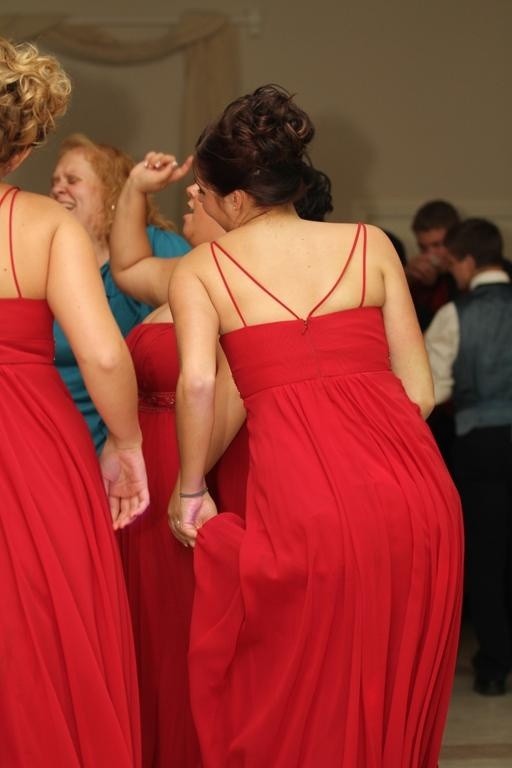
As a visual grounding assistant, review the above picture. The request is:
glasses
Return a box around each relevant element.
[418,241,443,253]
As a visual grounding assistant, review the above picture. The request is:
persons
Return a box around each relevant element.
[108,86,463,768]
[406,201,512,697]
[0,39,151,768]
[49,132,192,457]
